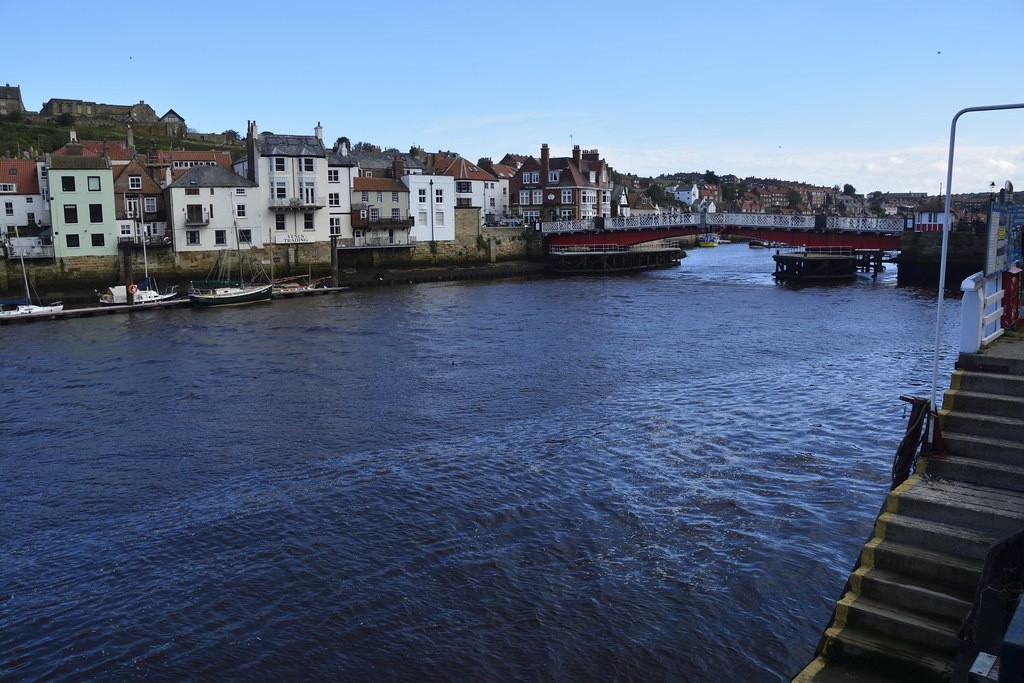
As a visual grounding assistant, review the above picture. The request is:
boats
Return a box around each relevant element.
[696,233,719,248]
[543,244,682,276]
[771,245,858,283]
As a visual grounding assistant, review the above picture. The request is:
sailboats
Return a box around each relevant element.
[0,226,64,318]
[99,193,179,307]
[187,192,272,308]
